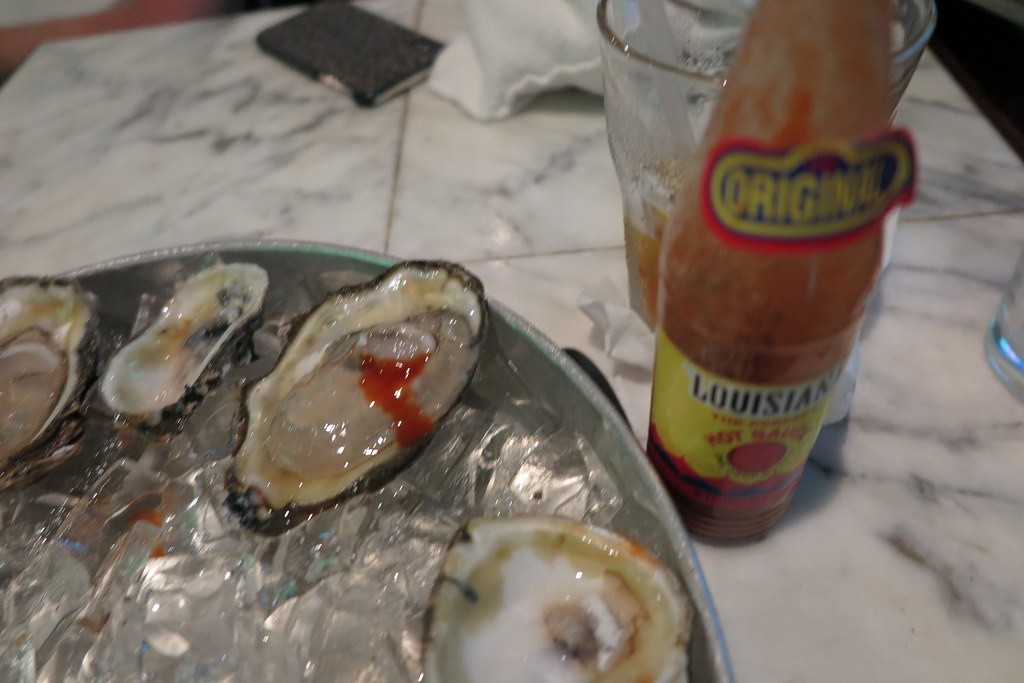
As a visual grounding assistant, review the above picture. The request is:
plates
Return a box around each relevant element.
[0,233,735,683]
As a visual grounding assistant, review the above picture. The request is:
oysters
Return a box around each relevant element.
[0,258,692,682]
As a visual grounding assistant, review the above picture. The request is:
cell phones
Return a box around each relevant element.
[256,0,447,104]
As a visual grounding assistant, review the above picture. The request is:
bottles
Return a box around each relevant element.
[646,0,884,545]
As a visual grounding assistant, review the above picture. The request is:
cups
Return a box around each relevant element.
[596,0,940,332]
[984,246,1024,406]
